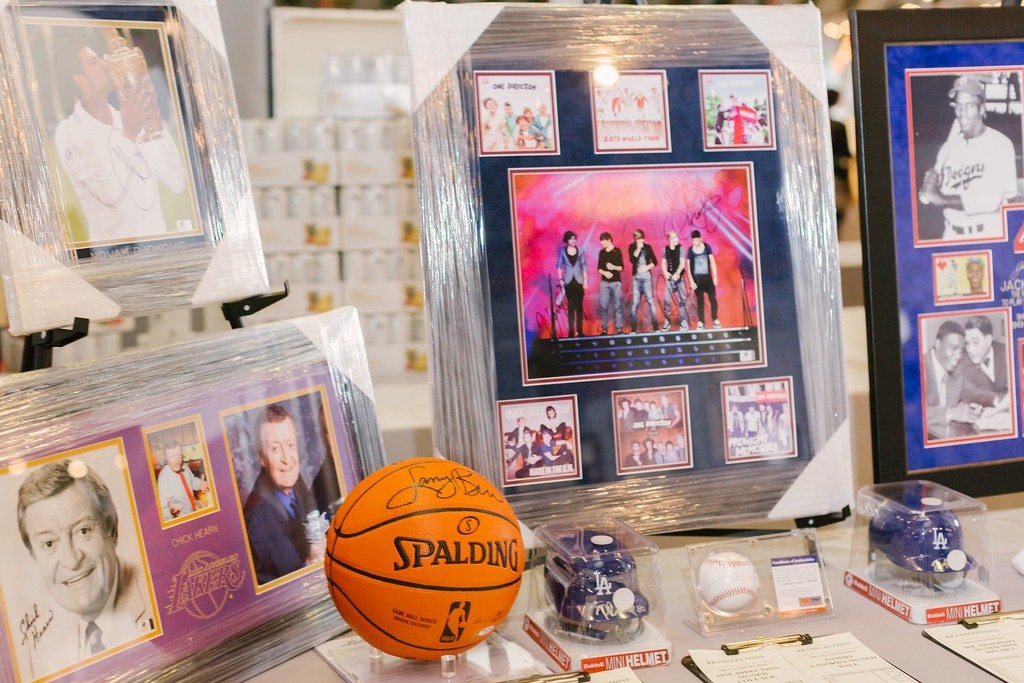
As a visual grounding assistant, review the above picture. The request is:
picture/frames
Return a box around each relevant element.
[847,6,1024,505]
[0,305,389,683]
[0,0,272,337]
[394,0,856,550]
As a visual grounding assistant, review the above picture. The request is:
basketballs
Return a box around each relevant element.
[326,458,524,664]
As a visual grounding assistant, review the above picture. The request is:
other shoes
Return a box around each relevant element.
[662,319,671,331]
[630,326,637,334]
[654,323,660,332]
[698,323,705,330]
[714,319,721,329]
[680,323,688,330]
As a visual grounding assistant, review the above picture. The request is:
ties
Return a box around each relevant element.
[178,471,196,511]
[85,622,105,655]
[291,499,305,524]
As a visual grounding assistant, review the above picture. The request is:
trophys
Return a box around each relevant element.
[102,25,165,141]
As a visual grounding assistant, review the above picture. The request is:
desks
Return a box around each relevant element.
[244,384,1024,683]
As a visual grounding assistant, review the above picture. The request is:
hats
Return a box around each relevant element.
[948,77,984,101]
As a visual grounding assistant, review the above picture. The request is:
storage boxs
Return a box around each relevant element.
[520,514,674,673]
[53,115,429,382]
[842,479,1002,627]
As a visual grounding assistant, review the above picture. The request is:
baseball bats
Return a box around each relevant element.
[920,116,962,205]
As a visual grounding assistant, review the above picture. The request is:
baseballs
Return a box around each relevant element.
[697,549,762,612]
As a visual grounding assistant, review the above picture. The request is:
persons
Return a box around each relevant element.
[960,314,1010,439]
[481,85,792,484]
[18,459,155,683]
[244,404,326,587]
[922,321,984,443]
[916,74,1018,238]
[52,40,191,243]
[156,438,211,523]
[965,256,986,295]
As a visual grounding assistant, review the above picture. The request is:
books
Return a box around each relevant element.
[971,406,1012,435]
